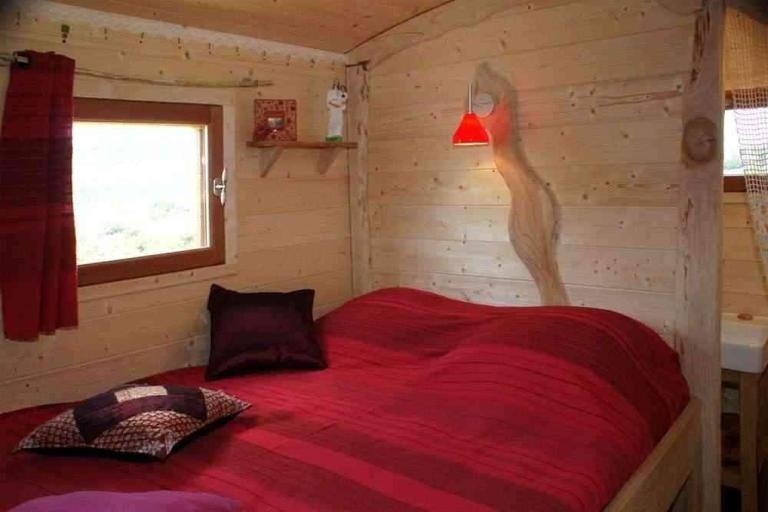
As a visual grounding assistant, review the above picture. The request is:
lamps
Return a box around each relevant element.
[452,80,490,146]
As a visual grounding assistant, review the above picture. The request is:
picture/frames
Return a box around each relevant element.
[254,99,298,143]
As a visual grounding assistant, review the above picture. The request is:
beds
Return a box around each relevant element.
[0,284,707,512]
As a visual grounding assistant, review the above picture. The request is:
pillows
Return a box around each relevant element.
[14,381,251,463]
[206,284,327,377]
